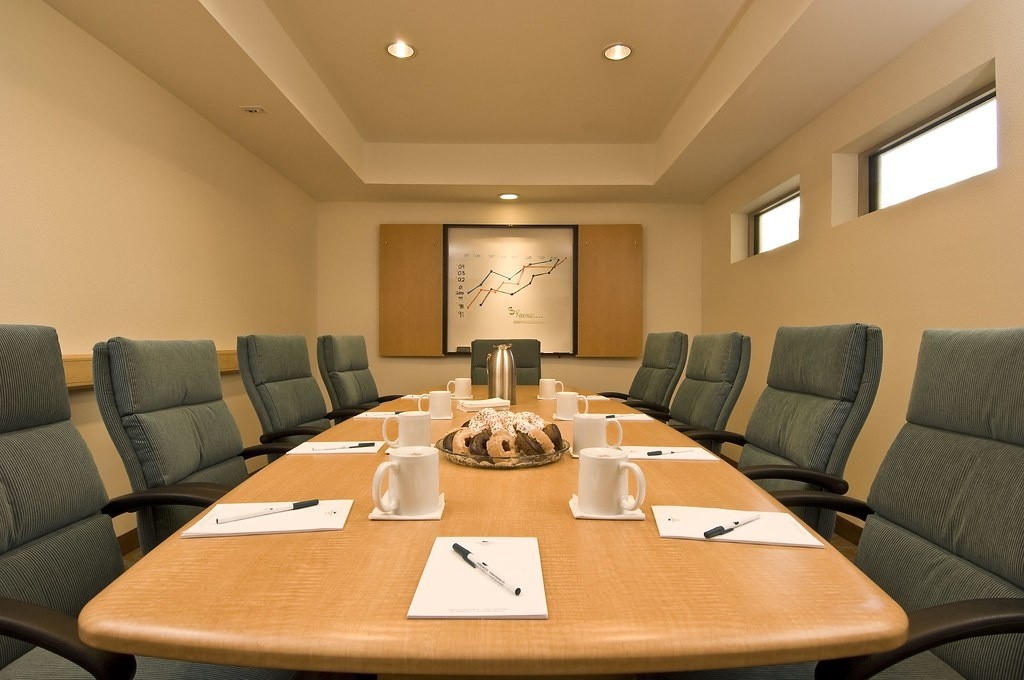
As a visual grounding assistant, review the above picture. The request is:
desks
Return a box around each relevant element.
[76,389,908,680]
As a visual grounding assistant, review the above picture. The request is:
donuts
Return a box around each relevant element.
[443,407,562,462]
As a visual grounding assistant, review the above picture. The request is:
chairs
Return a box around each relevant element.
[316,334,405,426]
[594,332,687,421]
[237,334,365,464]
[91,336,298,558]
[634,330,751,453]
[0,323,299,680]
[675,323,883,544]
[774,330,1024,680]
[470,338,542,387]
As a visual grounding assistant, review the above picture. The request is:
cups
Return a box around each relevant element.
[382,411,431,448]
[371,446,441,516]
[557,391,589,419]
[418,390,452,418]
[447,378,472,399]
[580,445,646,516]
[573,413,622,455]
[539,379,564,398]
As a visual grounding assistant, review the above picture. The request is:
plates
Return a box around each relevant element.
[435,436,571,469]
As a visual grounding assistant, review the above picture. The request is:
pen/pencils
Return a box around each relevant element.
[647,448,696,456]
[705,513,761,538]
[365,412,400,416]
[452,543,523,596]
[411,395,424,398]
[214,499,321,525]
[312,442,376,450]
[606,413,634,418]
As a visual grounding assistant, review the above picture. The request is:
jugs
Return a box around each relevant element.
[486,342,517,405]
